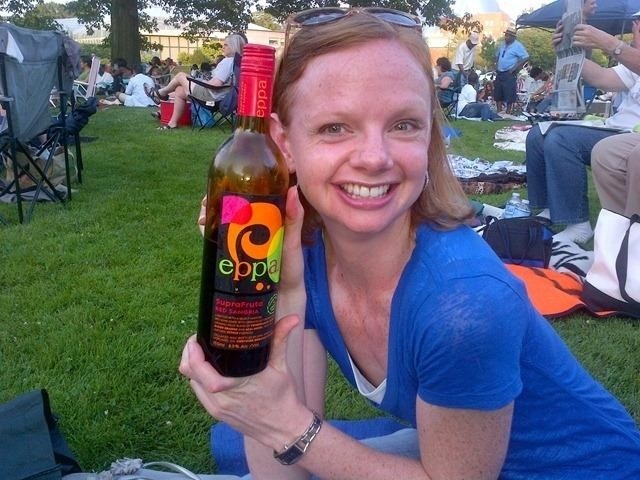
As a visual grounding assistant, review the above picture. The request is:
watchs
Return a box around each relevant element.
[611,41,625,59]
[272,406,323,465]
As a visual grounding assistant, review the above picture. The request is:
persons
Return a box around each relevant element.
[478,81,494,102]
[150,32,249,119]
[75,54,175,108]
[527,66,555,112]
[179,5,640,480]
[591,132,640,218]
[525,11,640,244]
[190,55,225,80]
[451,32,479,84]
[433,56,457,105]
[457,73,503,120]
[494,27,529,113]
[143,35,246,131]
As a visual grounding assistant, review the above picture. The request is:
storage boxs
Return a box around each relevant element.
[586,100,611,117]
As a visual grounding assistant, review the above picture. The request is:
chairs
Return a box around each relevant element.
[186,76,238,132]
[522,85,597,165]
[0,21,101,228]
[441,70,461,122]
[151,66,175,85]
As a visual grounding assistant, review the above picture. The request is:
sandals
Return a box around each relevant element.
[151,111,161,120]
[145,87,169,101]
[156,123,178,131]
[143,83,161,104]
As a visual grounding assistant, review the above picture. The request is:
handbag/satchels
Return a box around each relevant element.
[482,214,553,269]
[580,205,640,314]
[5,146,77,192]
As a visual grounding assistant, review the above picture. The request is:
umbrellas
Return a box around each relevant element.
[514,0,640,68]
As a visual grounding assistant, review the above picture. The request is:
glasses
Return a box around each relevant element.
[282,6,424,66]
[501,50,505,58]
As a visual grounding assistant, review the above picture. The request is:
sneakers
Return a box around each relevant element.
[551,220,594,245]
[535,208,551,220]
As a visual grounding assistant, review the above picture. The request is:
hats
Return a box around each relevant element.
[503,27,517,37]
[469,32,479,45]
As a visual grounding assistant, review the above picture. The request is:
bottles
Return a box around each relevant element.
[513,199,531,218]
[505,193,520,218]
[196,43,291,375]
